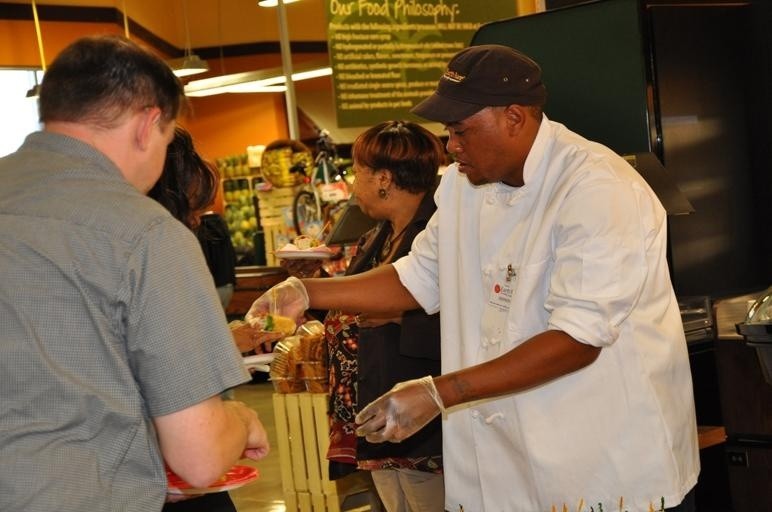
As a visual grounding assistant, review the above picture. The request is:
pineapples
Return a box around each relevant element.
[215,153,251,178]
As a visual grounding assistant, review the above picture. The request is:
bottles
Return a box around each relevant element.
[215,155,261,203]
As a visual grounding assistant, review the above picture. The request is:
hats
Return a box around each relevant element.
[408,43,549,124]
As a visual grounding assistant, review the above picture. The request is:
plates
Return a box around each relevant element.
[167,465,259,497]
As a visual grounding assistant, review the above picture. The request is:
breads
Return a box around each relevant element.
[270,320,329,392]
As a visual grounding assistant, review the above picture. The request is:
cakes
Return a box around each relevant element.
[279,236,330,251]
[252,313,296,334]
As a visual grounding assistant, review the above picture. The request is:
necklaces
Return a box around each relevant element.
[379,224,408,261]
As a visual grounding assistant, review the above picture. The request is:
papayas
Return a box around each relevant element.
[224,190,262,248]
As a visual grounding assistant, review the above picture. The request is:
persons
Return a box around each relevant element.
[323,119,447,512]
[143,120,237,511]
[1,35,270,511]
[244,42,705,512]
